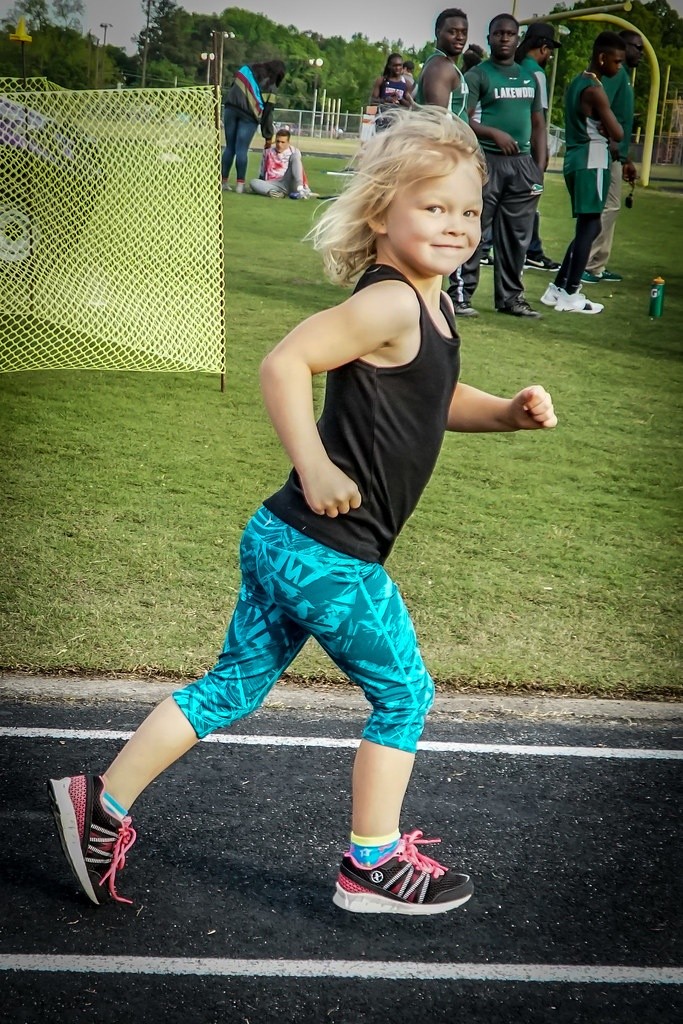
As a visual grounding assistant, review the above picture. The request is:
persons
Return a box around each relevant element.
[540,31,625,315]
[480,24,561,272]
[371,53,415,111]
[447,13,547,318]
[222,60,286,193]
[403,61,418,97]
[250,129,312,199]
[50,105,559,915]
[461,44,482,73]
[415,8,468,125]
[581,30,644,284]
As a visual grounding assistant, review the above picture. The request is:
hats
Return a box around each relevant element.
[272,60,286,87]
[525,23,562,50]
[402,61,414,69]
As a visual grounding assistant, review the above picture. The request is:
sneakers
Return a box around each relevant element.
[453,300,480,318]
[555,285,605,314]
[523,257,561,272]
[46,774,137,904]
[580,270,599,283]
[296,190,310,200]
[540,282,559,306]
[269,189,285,199]
[498,301,543,318]
[222,181,232,190]
[333,830,475,915]
[594,270,623,281]
[479,255,494,266]
[236,183,246,193]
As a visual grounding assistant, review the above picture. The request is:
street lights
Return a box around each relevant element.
[210,30,235,86]
[201,52,216,86]
[307,57,324,103]
[99,23,114,87]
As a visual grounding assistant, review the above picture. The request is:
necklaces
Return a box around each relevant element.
[584,70,601,83]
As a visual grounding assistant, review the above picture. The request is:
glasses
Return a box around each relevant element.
[628,42,645,52]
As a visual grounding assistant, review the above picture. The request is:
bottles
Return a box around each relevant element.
[649,276,665,317]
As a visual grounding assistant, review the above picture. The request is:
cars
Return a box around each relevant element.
[0,96,111,281]
